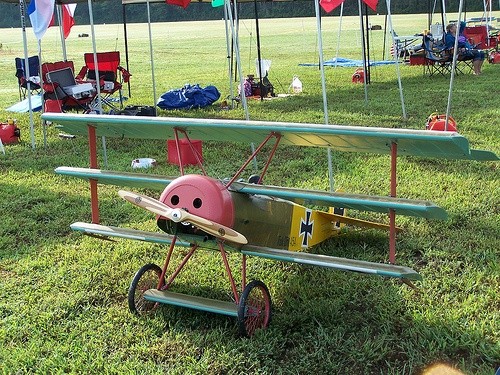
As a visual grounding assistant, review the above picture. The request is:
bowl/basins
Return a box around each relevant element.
[130,158,157,169]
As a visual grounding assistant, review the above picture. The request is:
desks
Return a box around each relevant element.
[395,35,427,61]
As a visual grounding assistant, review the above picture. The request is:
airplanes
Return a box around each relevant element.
[40,112,500,340]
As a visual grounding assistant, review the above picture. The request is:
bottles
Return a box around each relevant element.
[292,77,302,93]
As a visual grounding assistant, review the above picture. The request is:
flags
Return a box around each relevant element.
[62,4,78,39]
[212,0,225,7]
[27,0,55,41]
[319,0,343,13]
[165,0,190,8]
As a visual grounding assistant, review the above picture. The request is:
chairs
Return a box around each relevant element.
[15,52,133,116]
[421,30,500,79]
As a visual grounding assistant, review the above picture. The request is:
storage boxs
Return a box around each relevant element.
[168,139,202,164]
[410,54,433,65]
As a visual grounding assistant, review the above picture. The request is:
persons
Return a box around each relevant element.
[445,24,485,75]
